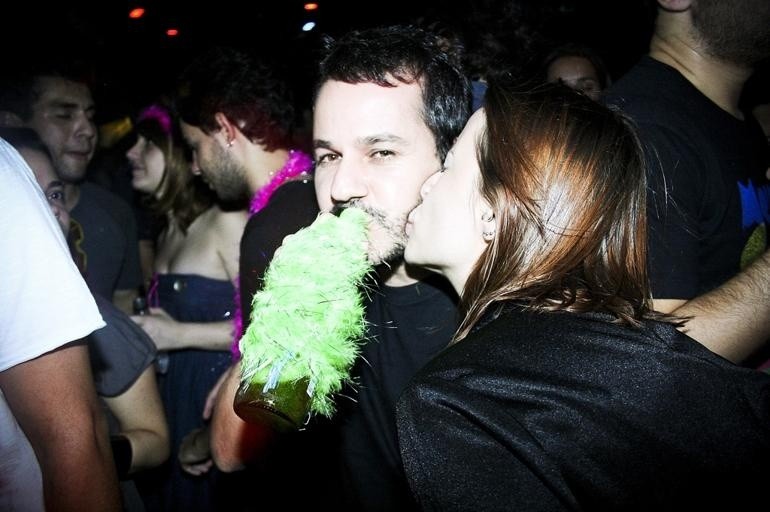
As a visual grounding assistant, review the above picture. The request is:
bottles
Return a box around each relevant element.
[132,297,170,375]
[232,375,314,438]
